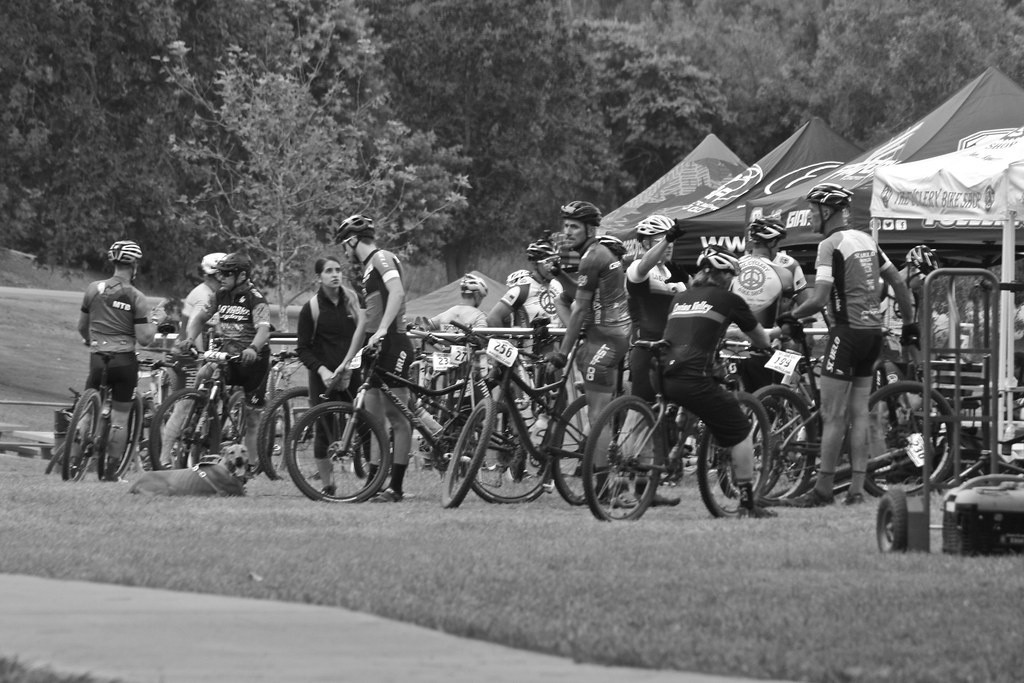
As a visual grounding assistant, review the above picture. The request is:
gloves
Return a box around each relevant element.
[900,323,919,346]
[775,312,798,328]
[666,218,687,242]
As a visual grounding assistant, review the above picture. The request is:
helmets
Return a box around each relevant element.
[526,238,562,261]
[595,235,628,255]
[460,274,488,298]
[506,270,532,288]
[805,183,854,207]
[108,240,143,263]
[906,245,941,275]
[696,245,741,276]
[201,253,227,275]
[636,214,675,242]
[560,200,602,226]
[216,253,252,279]
[747,218,787,244]
[335,215,375,244]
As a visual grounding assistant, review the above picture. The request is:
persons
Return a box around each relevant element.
[424,184,941,519]
[297,255,366,500]
[181,251,271,478]
[66,241,167,483]
[147,252,229,474]
[329,214,415,503]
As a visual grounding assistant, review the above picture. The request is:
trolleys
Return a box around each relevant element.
[876,268,1013,555]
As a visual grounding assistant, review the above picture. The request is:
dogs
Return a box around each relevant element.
[127,444,249,497]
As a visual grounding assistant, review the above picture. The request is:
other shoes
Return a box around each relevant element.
[635,479,682,506]
[737,508,779,521]
[611,493,636,509]
[842,492,866,507]
[780,489,835,509]
[320,487,336,499]
[102,474,130,483]
[508,448,526,483]
[370,489,403,503]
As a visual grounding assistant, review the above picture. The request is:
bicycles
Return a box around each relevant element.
[43,301,956,521]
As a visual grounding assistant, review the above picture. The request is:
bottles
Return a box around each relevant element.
[210,380,221,400]
[413,404,443,435]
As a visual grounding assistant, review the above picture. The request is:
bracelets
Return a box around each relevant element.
[248,344,258,352]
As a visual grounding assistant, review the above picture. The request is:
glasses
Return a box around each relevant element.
[341,234,358,251]
[221,270,236,277]
[537,256,561,265]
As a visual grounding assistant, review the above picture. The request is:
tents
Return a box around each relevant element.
[744,67,1024,440]
[606,115,865,270]
[549,133,751,273]
